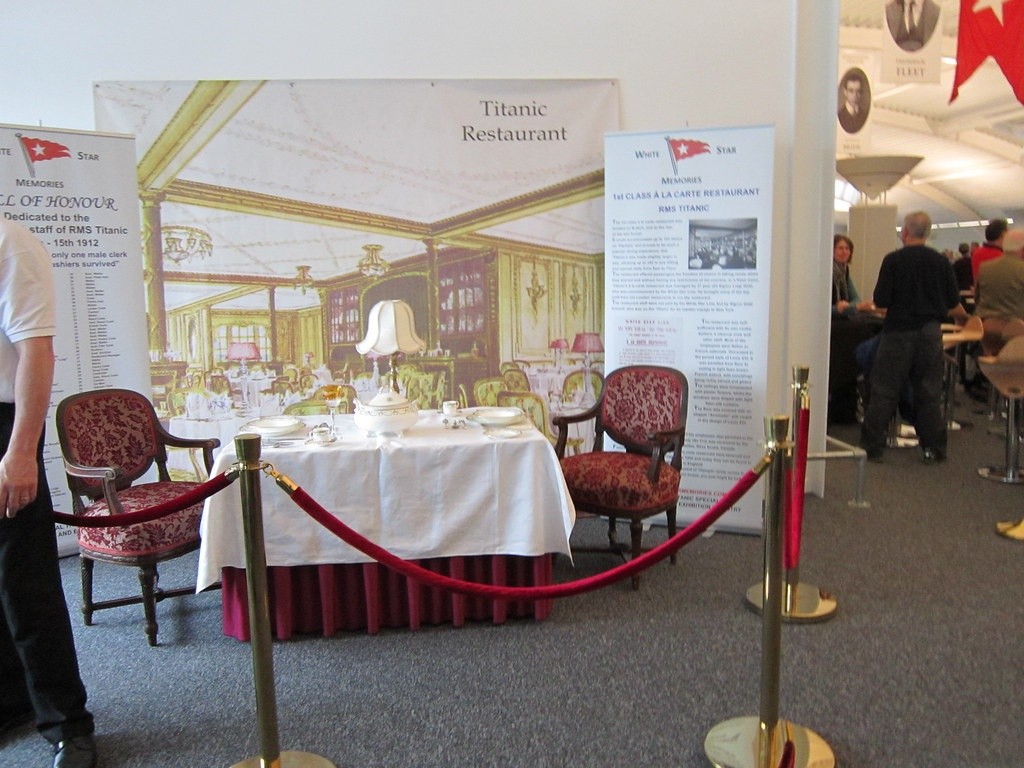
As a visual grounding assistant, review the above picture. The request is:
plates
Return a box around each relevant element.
[247,418,300,430]
[466,415,526,423]
[474,409,522,419]
[239,423,306,435]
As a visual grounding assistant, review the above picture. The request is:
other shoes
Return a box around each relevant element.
[868,450,884,463]
[924,445,947,465]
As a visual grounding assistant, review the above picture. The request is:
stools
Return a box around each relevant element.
[941,290,1024,484]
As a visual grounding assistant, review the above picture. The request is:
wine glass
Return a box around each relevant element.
[323,385,342,437]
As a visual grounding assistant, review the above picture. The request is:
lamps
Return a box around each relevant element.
[224,341,261,406]
[140,225,214,266]
[291,266,314,296]
[548,338,570,373]
[571,332,606,411]
[351,298,427,440]
[356,242,390,277]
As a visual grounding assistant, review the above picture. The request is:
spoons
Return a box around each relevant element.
[443,418,449,429]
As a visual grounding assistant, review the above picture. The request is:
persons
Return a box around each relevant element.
[886,0,940,51]
[0,215,95,768]
[689,234,758,269]
[833,213,1024,464]
[838,75,865,132]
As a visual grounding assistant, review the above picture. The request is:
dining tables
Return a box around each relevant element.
[169,409,251,478]
[292,366,332,386]
[320,379,421,404]
[223,369,277,402]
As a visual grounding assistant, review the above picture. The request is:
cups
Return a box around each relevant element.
[443,401,459,415]
[309,426,330,445]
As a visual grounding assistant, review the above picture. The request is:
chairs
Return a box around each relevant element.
[460,358,585,459]
[552,366,690,587]
[167,364,395,414]
[56,389,221,646]
[563,369,607,457]
[406,369,446,413]
[195,407,578,645]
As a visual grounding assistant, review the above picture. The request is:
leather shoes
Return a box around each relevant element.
[52,731,97,768]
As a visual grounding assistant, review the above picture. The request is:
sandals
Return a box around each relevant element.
[995,519,1024,542]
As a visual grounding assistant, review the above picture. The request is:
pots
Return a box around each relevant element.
[352,383,419,436]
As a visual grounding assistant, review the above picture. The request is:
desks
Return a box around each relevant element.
[452,357,489,408]
[844,307,921,451]
